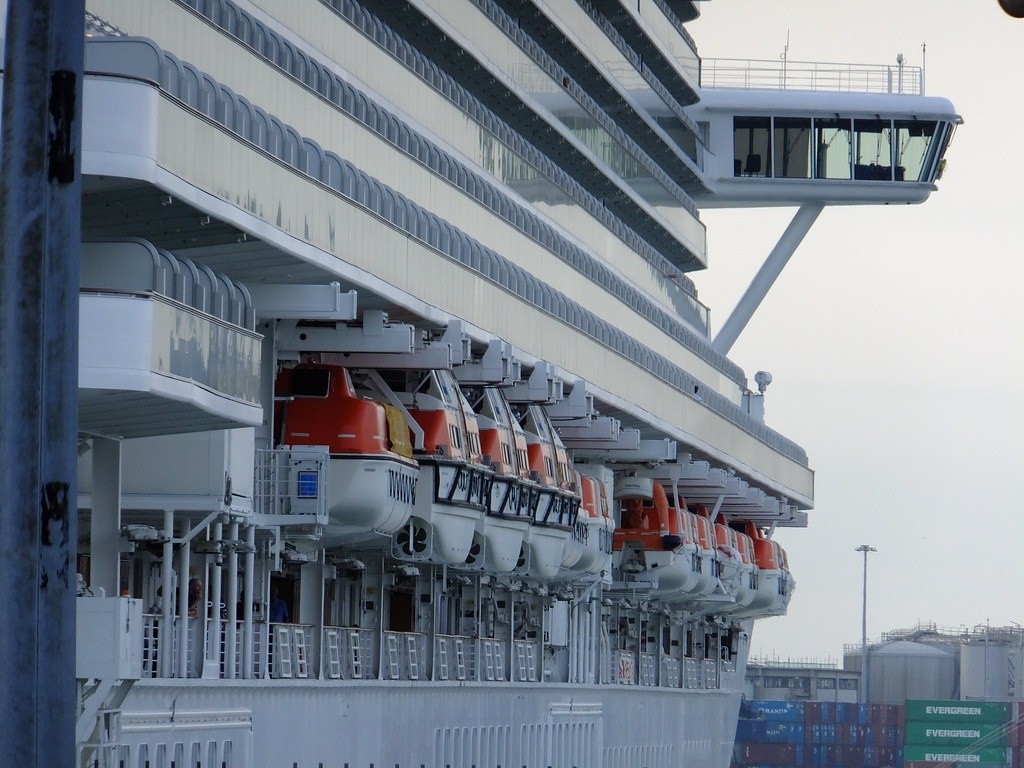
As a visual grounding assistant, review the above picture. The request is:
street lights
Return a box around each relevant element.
[856,546,879,701]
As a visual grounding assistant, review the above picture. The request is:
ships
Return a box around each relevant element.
[0,0,963,766]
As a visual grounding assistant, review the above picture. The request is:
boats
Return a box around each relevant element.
[464,384,540,579]
[613,480,796,623]
[553,472,615,598]
[358,369,498,570]
[513,403,582,586]
[272,367,420,554]
